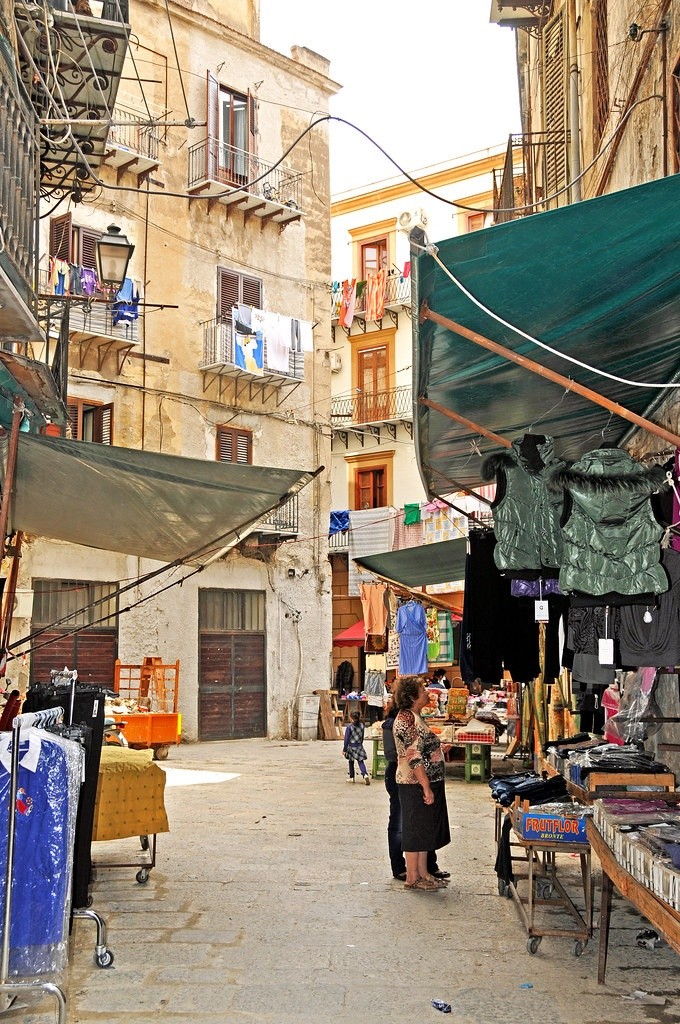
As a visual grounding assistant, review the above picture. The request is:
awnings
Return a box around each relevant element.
[350,530,468,619]
[332,604,463,648]
[5,427,321,659]
[408,172,680,531]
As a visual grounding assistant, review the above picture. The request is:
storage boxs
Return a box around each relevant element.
[510,807,590,845]
[423,686,495,744]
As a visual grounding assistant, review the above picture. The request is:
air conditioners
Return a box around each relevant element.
[330,352,342,372]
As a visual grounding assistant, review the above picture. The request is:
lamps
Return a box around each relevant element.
[39,221,136,317]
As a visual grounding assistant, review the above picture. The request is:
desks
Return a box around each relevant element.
[340,699,368,726]
[372,737,487,783]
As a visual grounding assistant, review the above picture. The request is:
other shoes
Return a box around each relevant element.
[428,869,450,878]
[363,774,370,785]
[346,777,355,783]
[395,873,407,881]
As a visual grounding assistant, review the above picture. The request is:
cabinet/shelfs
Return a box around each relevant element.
[492,755,680,983]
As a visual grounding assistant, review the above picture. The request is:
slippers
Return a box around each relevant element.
[404,872,448,891]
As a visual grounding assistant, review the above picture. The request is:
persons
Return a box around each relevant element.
[430,668,451,689]
[368,705,384,726]
[343,710,370,785]
[383,673,451,890]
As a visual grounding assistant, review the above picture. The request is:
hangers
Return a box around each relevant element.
[28,669,104,697]
[5,706,82,741]
[599,428,618,449]
